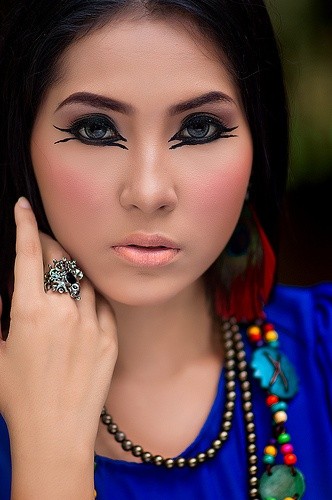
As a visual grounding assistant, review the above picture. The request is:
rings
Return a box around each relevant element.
[44,257,84,301]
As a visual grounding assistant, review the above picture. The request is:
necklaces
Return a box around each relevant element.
[100,318,259,500]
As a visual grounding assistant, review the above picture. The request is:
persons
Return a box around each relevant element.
[0,0,332,500]
